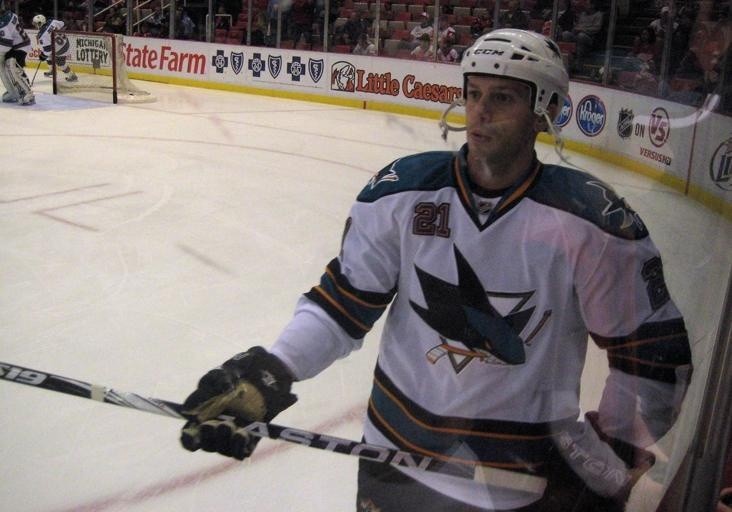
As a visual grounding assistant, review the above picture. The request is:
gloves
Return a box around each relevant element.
[514,410,657,512]
[179,346,297,462]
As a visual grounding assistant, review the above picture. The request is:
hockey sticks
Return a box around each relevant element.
[29,60,42,87]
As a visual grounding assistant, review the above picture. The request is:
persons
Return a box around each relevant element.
[178,26,692,511]
[63,0,386,55]
[0,10,36,106]
[410,0,732,112]
[32,15,77,81]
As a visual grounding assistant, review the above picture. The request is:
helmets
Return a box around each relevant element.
[31,14,47,28]
[460,26,570,117]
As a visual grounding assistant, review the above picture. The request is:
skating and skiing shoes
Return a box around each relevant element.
[65,73,78,82]
[2,92,18,101]
[17,92,35,104]
[44,70,56,78]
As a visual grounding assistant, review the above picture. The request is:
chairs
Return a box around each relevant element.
[1,0,732,112]
